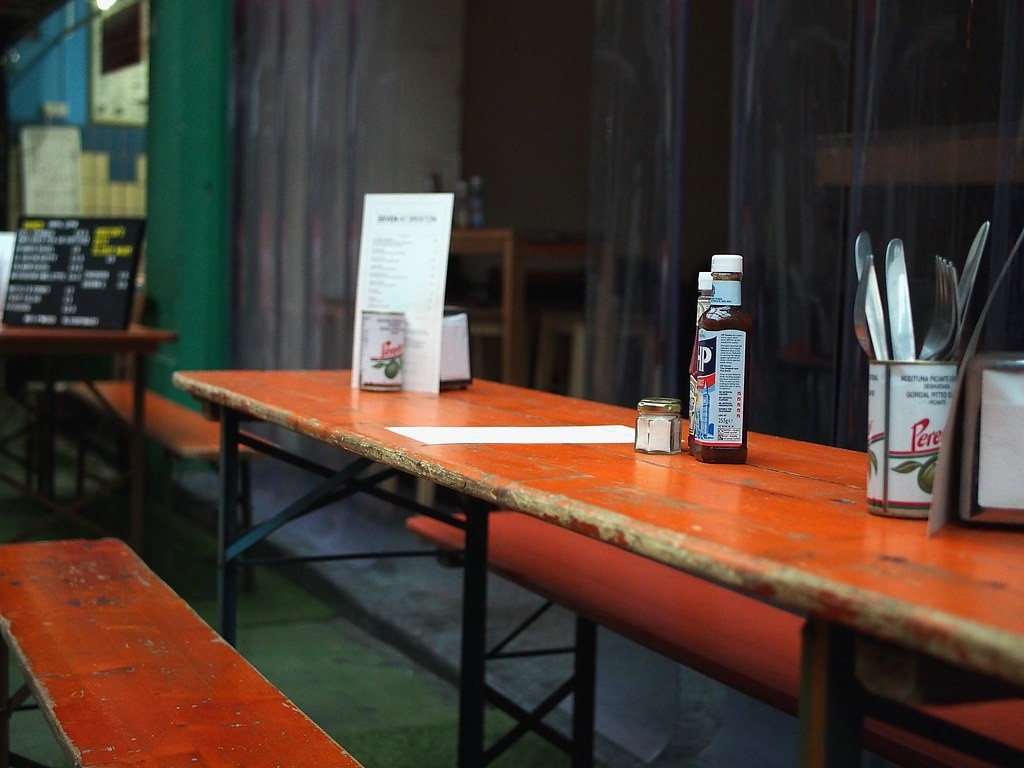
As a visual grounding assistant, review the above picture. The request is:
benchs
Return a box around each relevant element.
[60,380,277,462]
[0,543,372,768]
[404,507,1024,768]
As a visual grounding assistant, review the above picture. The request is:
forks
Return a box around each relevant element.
[931,262,961,360]
[853,254,876,360]
[918,253,953,360]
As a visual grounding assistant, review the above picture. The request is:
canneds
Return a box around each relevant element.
[359,310,406,392]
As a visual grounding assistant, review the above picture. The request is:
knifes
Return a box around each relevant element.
[854,230,889,361]
[959,222,991,325]
[885,238,917,361]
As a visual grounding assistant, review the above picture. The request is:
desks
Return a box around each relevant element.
[0,323,177,555]
[175,359,1024,768]
[452,224,596,386]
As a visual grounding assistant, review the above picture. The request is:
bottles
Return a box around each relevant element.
[693,255,752,464]
[688,272,712,458]
[634,398,684,455]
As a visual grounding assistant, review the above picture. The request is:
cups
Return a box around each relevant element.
[864,360,959,520]
[358,309,406,392]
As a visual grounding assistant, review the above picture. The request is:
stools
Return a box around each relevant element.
[534,310,664,407]
